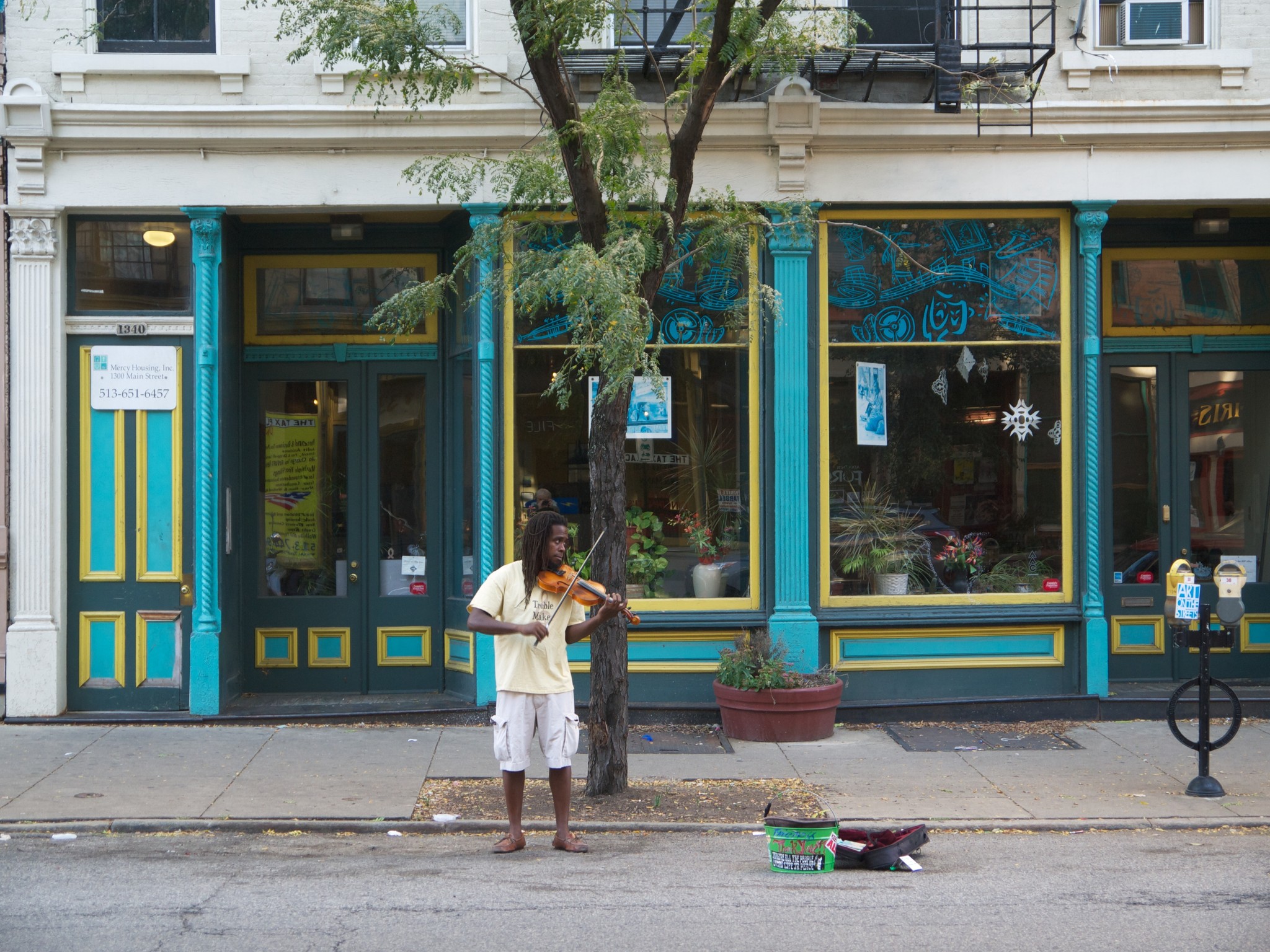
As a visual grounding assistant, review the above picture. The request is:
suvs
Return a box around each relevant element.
[683,503,961,598]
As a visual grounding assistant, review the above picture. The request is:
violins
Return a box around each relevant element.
[538,564,641,626]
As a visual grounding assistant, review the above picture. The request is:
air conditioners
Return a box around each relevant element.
[1116,0,1191,47]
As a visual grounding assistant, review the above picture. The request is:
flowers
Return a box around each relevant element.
[931,526,987,573]
[669,512,731,564]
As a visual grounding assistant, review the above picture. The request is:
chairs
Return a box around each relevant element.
[982,537,1000,554]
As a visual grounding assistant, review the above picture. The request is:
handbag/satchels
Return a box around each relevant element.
[876,420,885,435]
[865,409,884,431]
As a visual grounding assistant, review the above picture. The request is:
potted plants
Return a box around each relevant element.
[713,629,845,742]
[516,422,750,597]
[832,476,1045,595]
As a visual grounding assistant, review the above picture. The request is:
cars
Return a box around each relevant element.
[1034,540,1234,592]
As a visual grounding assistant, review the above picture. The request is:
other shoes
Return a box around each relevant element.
[860,413,868,421]
[552,833,589,852]
[492,834,526,852]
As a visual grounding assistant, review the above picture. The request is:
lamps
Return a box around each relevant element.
[329,214,364,242]
[141,220,177,248]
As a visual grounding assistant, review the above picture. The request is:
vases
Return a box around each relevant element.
[692,563,722,598]
[951,572,969,592]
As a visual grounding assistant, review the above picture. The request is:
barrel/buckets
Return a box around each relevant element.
[763,790,841,874]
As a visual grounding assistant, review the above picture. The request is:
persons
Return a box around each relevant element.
[467,512,629,854]
[525,488,560,518]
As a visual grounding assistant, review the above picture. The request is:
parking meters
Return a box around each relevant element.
[1162,557,1244,797]
